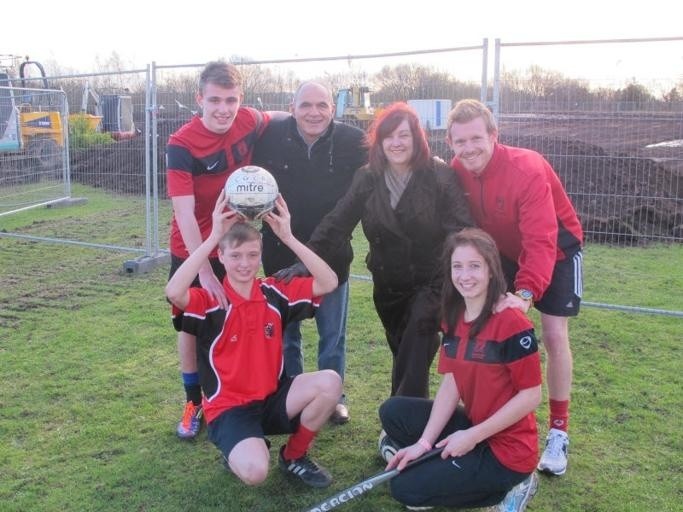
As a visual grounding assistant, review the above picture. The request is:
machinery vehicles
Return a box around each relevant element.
[334,84,381,124]
[78,85,136,140]
[0,59,103,186]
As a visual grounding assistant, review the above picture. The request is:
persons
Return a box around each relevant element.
[269,101,476,397]
[442,96,584,477]
[164,188,344,488]
[162,61,292,442]
[378,226,542,511]
[248,73,372,425]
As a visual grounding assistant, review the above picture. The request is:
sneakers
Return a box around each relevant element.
[494,472,537,512]
[536,428,569,476]
[177,401,202,438]
[331,404,350,423]
[278,445,333,488]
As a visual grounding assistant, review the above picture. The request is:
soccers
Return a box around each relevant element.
[224,165,277,221]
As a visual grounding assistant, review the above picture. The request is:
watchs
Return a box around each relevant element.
[514,288,535,309]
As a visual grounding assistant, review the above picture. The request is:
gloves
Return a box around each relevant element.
[273,262,309,285]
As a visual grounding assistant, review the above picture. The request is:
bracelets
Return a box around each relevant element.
[415,438,433,452]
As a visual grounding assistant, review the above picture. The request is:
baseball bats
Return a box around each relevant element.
[306,445,447,511]
